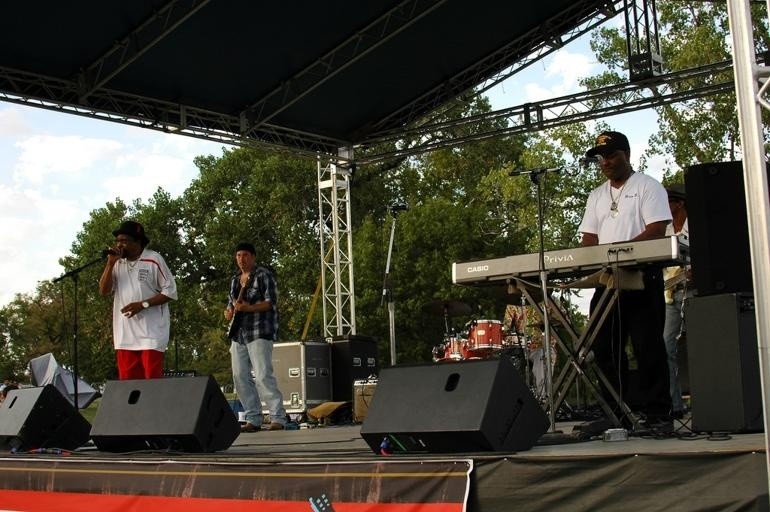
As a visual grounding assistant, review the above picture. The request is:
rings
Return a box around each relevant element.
[128,311,132,315]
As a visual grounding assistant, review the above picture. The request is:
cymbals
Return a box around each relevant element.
[497,281,553,305]
[422,300,475,316]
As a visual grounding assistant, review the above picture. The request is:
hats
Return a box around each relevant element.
[666,183,686,199]
[113,221,150,244]
[586,131,629,158]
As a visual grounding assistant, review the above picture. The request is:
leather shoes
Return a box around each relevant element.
[271,422,282,430]
[241,423,261,432]
[672,410,684,419]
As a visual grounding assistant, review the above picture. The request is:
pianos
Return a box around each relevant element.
[451,235,680,286]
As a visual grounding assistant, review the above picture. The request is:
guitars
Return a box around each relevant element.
[226,274,253,341]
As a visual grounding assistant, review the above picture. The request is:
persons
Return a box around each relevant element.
[222,243,288,433]
[661,181,698,418]
[501,294,561,401]
[0,378,22,405]
[571,130,673,438]
[98,220,179,381]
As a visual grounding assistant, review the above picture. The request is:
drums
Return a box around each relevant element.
[468,319,502,350]
[460,339,491,359]
[444,335,461,361]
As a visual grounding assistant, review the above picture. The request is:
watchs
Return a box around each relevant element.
[141,300,150,310]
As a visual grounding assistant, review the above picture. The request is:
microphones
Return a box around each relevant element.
[580,156,601,161]
[94,250,117,256]
[389,204,410,210]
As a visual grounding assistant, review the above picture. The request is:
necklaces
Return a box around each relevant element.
[609,171,629,211]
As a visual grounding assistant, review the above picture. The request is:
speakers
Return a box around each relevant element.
[0,385,92,451]
[359,356,554,452]
[684,162,769,297]
[89,374,241,453]
[685,293,763,434]
